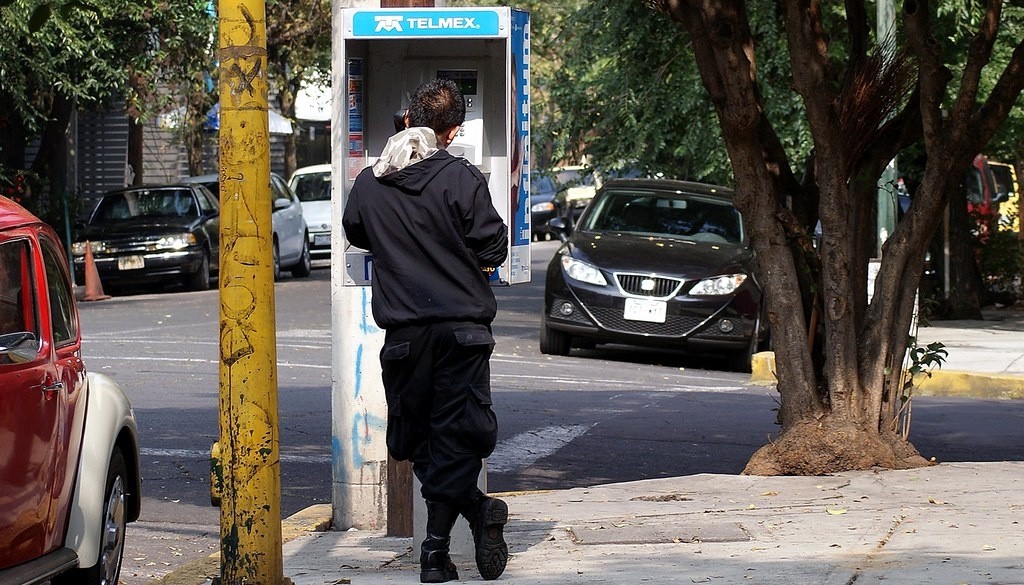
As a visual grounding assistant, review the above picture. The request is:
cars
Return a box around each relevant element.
[550,164,604,225]
[0,194,144,585]
[175,171,312,280]
[71,184,220,293]
[896,157,1022,260]
[529,171,570,238]
[608,159,685,179]
[540,177,773,372]
[285,163,331,256]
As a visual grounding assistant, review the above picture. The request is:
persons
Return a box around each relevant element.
[342,78,509,583]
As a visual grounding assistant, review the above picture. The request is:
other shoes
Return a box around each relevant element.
[470,496,507,580]
[421,536,458,583]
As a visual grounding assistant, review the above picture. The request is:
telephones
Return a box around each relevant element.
[393,109,406,134]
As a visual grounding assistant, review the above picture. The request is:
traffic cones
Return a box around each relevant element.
[78,239,112,301]
